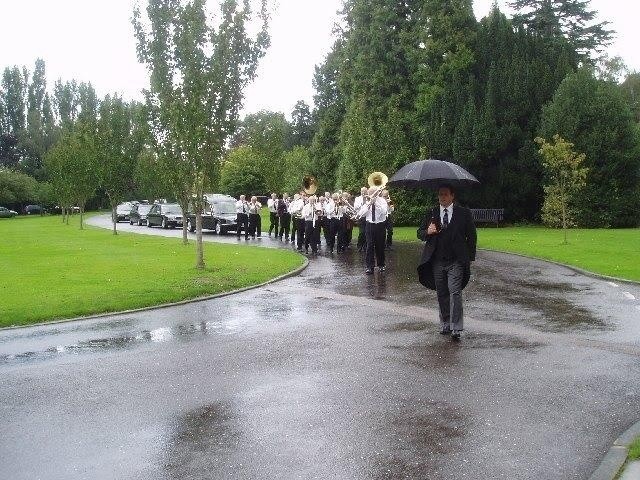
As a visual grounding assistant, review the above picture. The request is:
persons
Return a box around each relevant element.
[416,183,477,342]
[235,186,395,275]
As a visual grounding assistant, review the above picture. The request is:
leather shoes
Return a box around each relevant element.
[440,326,450,333]
[365,267,373,274]
[452,330,460,339]
[380,266,385,272]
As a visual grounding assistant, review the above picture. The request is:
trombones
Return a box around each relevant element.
[312,197,321,228]
[356,189,381,220]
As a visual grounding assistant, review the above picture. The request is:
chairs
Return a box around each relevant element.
[470,209,505,228]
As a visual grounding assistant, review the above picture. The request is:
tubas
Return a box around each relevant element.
[299,174,317,196]
[368,171,388,191]
[339,194,356,221]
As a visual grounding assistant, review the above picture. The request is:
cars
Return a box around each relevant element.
[0,206,20,218]
[47,203,80,214]
[111,204,131,222]
[129,204,151,226]
[122,198,166,204]
[149,202,184,229]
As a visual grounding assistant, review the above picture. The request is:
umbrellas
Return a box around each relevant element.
[385,159,482,234]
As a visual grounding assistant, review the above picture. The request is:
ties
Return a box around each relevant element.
[443,208,449,228]
[372,203,375,221]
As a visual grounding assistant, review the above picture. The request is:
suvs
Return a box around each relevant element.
[23,205,48,215]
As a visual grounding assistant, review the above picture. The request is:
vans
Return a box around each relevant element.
[186,192,250,234]
